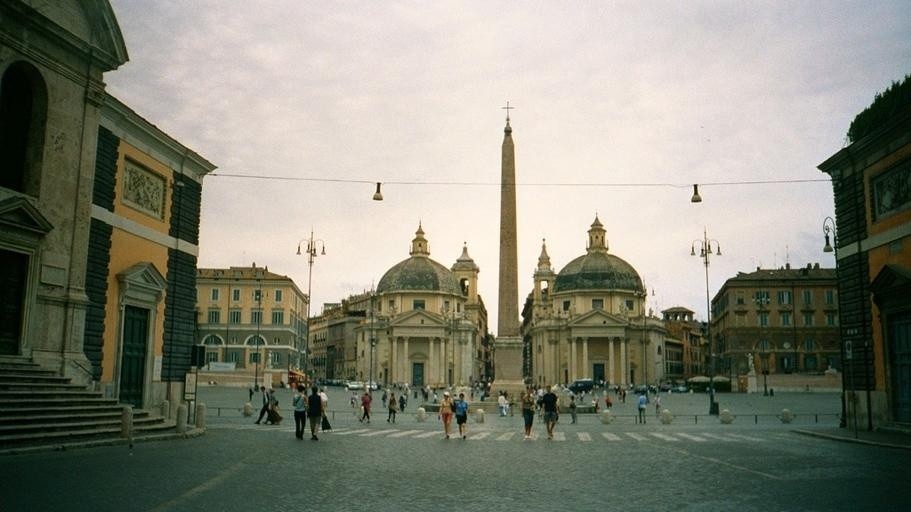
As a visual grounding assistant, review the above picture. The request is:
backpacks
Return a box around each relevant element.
[321,416,331,431]
[275,400,279,407]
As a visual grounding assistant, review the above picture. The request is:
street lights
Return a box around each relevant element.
[298,230,326,393]
[821,214,848,430]
[251,284,269,392]
[691,225,723,416]
[751,289,774,397]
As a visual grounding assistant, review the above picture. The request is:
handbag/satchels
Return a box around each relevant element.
[463,410,467,420]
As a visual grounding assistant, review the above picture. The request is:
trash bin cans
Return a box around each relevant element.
[713,401,719,415]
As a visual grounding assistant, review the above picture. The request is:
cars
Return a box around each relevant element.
[634,381,686,394]
[322,377,377,391]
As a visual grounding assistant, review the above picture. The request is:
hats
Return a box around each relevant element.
[442,390,451,396]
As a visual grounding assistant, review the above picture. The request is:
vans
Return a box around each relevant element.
[569,379,593,393]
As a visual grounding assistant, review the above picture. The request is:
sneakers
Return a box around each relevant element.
[387,418,396,423]
[294,431,319,441]
[359,418,371,424]
[255,421,280,425]
[442,434,468,441]
[523,433,555,441]
